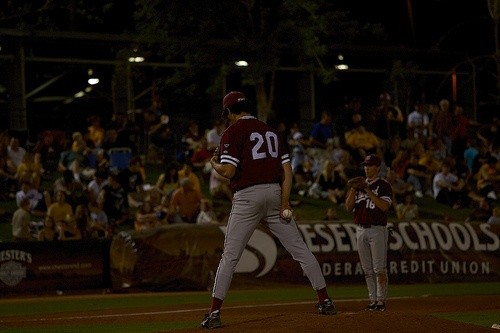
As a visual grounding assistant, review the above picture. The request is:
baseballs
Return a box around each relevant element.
[283,209,292,218]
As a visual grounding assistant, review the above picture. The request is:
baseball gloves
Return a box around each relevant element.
[347,177,366,189]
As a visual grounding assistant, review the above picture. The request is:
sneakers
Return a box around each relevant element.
[377,302,385,311]
[200,311,222,328]
[367,302,377,311]
[316,298,335,315]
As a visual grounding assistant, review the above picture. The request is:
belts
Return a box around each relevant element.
[356,222,386,227]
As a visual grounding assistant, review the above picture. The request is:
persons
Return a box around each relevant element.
[11,198,35,242]
[429,99,452,135]
[207,119,226,148]
[156,163,179,199]
[127,184,145,217]
[446,153,471,178]
[1,153,18,196]
[107,112,137,154]
[345,154,393,310]
[363,133,449,177]
[7,135,27,165]
[140,110,174,151]
[51,220,83,240]
[200,90,337,328]
[101,173,129,224]
[141,144,167,165]
[84,202,108,238]
[14,181,49,210]
[285,122,311,160]
[464,198,500,224]
[135,202,157,229]
[47,190,73,223]
[152,196,170,225]
[321,207,338,220]
[96,175,132,222]
[447,118,498,156]
[37,216,56,241]
[310,110,334,147]
[396,191,420,219]
[450,105,469,134]
[338,151,356,180]
[147,186,161,204]
[75,205,88,231]
[372,91,403,121]
[124,156,145,189]
[312,158,336,202]
[15,151,41,186]
[37,115,117,171]
[387,172,414,210]
[325,138,344,160]
[86,171,107,205]
[209,164,234,202]
[169,177,208,223]
[52,170,82,203]
[408,99,428,134]
[431,163,468,207]
[184,118,216,166]
[344,114,380,146]
[475,157,500,198]
[178,160,200,195]
[290,132,307,188]
[198,199,216,222]
[341,94,372,130]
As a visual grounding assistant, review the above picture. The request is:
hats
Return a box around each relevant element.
[361,154,381,166]
[222,91,248,120]
